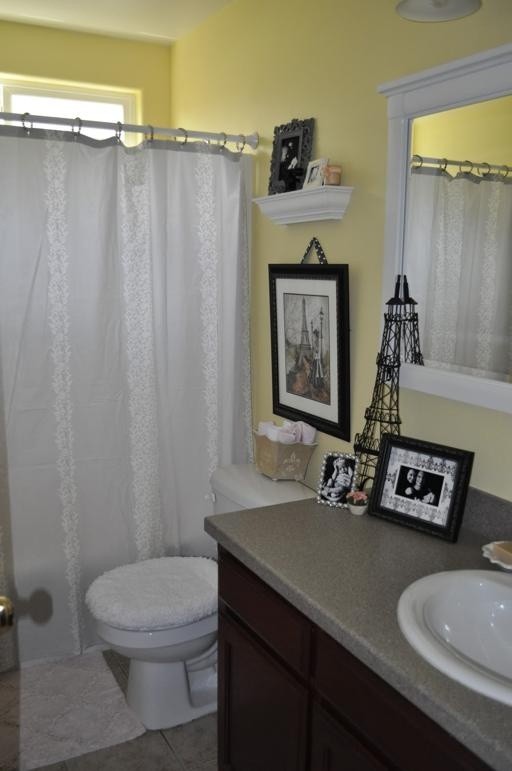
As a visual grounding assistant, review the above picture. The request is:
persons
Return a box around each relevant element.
[284,140,298,170]
[321,458,354,503]
[409,472,436,505]
[402,469,416,496]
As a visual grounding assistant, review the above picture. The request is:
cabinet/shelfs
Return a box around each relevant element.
[220,547,490,770]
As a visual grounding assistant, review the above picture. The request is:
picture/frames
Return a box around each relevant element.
[267,117,328,195]
[366,432,475,545]
[317,452,358,508]
[266,263,351,443]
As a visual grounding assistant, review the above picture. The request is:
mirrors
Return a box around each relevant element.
[379,38,511,413]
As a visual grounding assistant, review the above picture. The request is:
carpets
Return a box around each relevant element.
[18,651,147,771]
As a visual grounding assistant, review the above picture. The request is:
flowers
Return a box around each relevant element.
[346,490,370,505]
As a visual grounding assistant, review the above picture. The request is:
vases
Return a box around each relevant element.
[347,503,368,516]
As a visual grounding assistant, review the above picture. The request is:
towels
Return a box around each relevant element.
[257,420,316,448]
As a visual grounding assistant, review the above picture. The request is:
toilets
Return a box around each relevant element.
[83,467,318,734]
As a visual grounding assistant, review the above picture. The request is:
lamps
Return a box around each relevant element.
[393,0,483,27]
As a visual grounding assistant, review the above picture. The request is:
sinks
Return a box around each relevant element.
[396,566,512,710]
